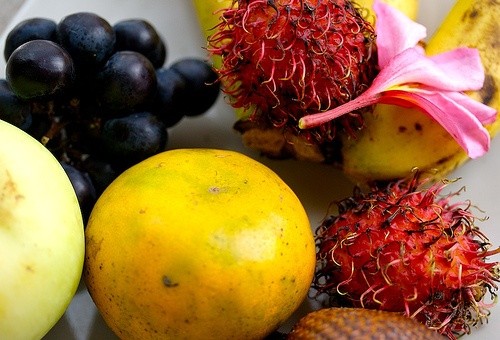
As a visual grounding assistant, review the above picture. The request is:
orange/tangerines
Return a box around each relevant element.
[85,148,316,340]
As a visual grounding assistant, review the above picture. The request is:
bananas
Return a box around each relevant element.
[191,0,500,186]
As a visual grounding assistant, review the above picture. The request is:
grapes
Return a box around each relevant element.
[0,10,222,218]
